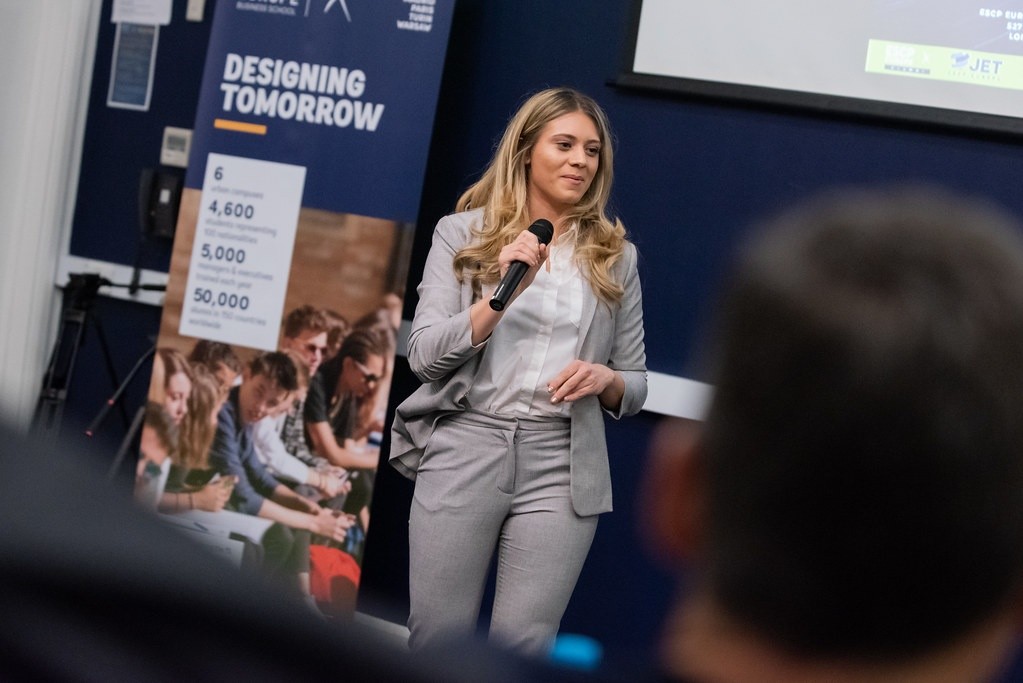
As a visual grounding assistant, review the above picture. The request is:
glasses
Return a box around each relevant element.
[353,359,383,383]
[291,337,329,356]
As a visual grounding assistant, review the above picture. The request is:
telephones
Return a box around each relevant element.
[137,166,185,249]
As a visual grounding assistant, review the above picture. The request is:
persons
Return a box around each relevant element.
[134,297,404,627]
[618,178,1023,683]
[388,84,647,665]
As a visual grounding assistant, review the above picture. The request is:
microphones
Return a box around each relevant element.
[489,218,554,312]
